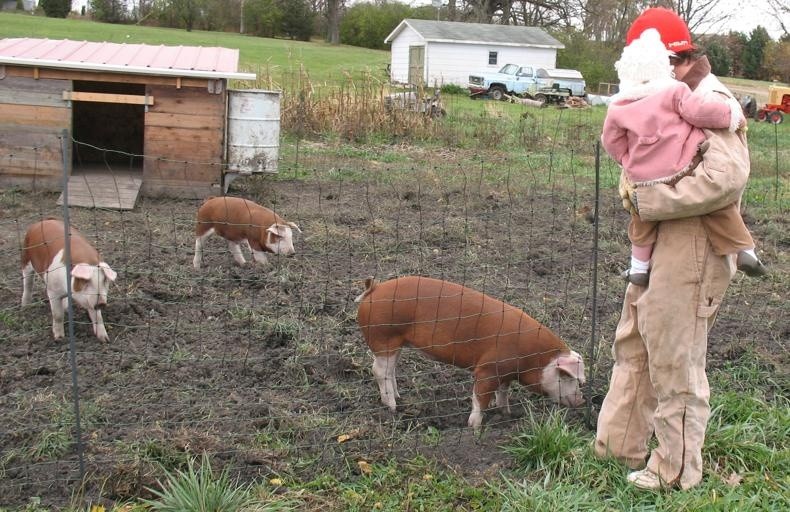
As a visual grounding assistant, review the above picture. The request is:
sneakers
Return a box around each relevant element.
[737,250,767,278]
[625,469,675,492]
[619,268,649,287]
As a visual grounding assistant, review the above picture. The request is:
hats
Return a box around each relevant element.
[615,29,672,84]
[627,7,697,51]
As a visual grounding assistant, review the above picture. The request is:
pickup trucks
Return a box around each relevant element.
[468,63,586,107]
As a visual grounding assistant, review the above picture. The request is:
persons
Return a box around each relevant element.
[575,7,751,491]
[600,28,765,286]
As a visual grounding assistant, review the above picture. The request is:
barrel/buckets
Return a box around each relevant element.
[226,90,282,173]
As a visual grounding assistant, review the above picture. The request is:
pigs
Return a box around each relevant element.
[192,194,302,270]
[18,216,119,344]
[353,273,587,434]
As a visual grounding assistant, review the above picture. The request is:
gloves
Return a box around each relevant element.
[618,171,637,216]
